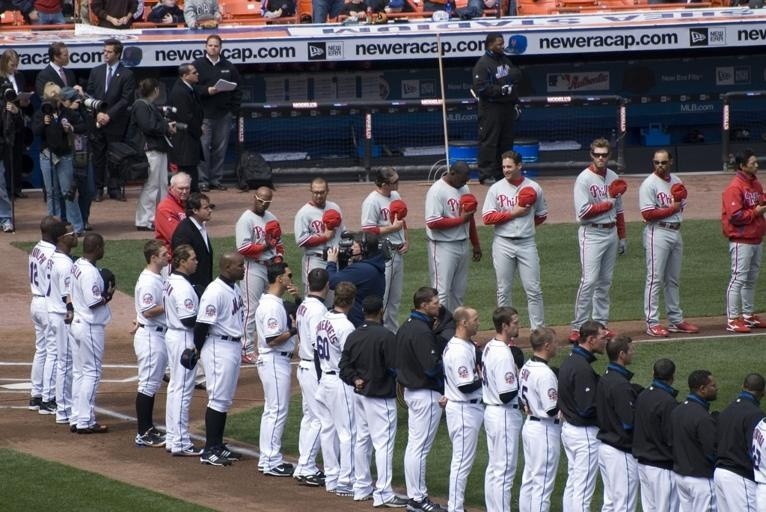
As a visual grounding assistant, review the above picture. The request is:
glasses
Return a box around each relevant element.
[255,195,272,205]
[57,232,75,240]
[654,160,668,165]
[591,152,609,158]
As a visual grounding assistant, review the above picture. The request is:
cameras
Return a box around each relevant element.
[42,100,57,113]
[170,120,187,133]
[1,82,16,102]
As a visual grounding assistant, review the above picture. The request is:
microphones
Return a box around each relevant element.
[351,251,362,257]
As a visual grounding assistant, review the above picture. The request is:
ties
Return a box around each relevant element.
[107,66,113,90]
[58,68,68,87]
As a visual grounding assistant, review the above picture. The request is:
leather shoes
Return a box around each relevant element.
[94,186,126,200]
[201,182,226,191]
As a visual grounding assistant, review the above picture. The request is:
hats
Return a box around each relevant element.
[460,193,476,216]
[265,220,282,241]
[390,201,406,224]
[324,209,341,230]
[672,182,688,202]
[610,180,627,198]
[519,186,537,207]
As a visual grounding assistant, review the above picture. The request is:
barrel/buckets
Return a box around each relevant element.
[447,140,482,181]
[513,136,538,178]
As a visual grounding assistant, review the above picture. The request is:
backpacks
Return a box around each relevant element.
[234,151,274,190]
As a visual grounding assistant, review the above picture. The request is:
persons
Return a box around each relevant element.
[1,0,766,511]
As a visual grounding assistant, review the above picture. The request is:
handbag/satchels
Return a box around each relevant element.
[104,140,150,182]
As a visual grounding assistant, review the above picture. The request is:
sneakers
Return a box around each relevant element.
[134,427,242,467]
[242,352,258,365]
[745,316,766,328]
[668,322,699,332]
[647,326,668,336]
[0,218,14,231]
[29,397,108,434]
[570,330,580,343]
[259,461,446,512]
[606,330,617,340]
[726,319,751,332]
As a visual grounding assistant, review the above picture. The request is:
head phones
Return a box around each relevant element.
[361,232,368,257]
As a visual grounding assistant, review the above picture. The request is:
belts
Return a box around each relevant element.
[496,404,519,410]
[139,324,169,332]
[504,236,525,239]
[591,223,617,228]
[281,351,293,358]
[471,398,482,404]
[326,371,336,375]
[658,222,681,230]
[208,333,241,342]
[316,253,322,256]
[529,416,560,424]
